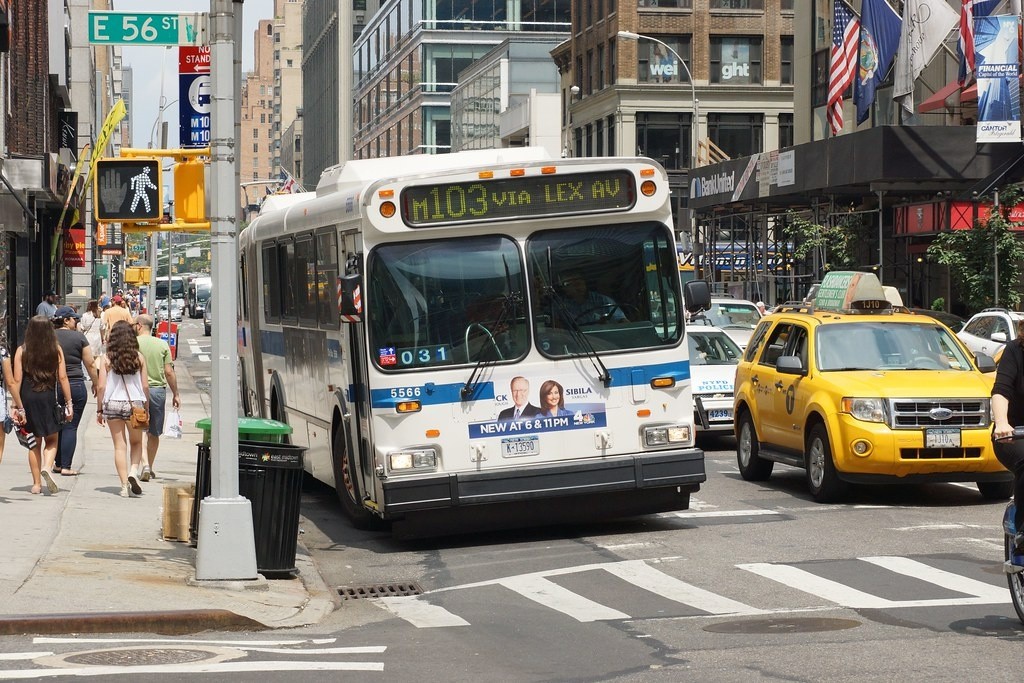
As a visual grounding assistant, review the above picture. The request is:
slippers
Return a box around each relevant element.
[53,469,61,473]
[62,470,80,475]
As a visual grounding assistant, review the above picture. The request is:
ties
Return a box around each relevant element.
[514,409,520,420]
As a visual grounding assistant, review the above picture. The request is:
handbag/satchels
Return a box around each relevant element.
[16,427,36,449]
[53,403,68,424]
[164,406,181,439]
[3,417,13,433]
[130,407,150,429]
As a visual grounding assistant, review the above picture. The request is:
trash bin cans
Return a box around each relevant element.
[200,440,303,580]
[193,416,291,537]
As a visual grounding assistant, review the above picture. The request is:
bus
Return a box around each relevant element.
[239,144,713,542]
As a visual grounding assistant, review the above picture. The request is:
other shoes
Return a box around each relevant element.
[150,470,155,479]
[41,468,58,493]
[128,473,142,495]
[31,485,40,493]
[1010,534,1024,565]
[140,465,150,482]
[119,490,129,497]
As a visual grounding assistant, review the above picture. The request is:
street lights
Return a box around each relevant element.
[616,29,701,280]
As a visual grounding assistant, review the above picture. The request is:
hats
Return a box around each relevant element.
[111,296,122,302]
[52,306,81,321]
[47,290,57,295]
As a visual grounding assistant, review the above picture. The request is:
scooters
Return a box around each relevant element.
[993,425,1024,625]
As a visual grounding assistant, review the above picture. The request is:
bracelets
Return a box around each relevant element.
[97,410,103,413]
[11,406,18,409]
[19,408,24,411]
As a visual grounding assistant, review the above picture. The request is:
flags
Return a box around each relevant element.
[956,0,1004,88]
[892,0,961,121]
[266,187,272,195]
[278,170,298,193]
[826,0,861,136]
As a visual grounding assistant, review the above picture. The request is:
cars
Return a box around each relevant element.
[155,271,212,337]
[731,269,1016,504]
[651,283,969,445]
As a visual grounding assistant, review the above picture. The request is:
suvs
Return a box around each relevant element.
[956,307,1024,363]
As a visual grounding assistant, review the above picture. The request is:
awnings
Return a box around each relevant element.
[960,74,1024,103]
[918,79,962,114]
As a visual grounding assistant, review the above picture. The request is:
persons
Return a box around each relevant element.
[498,376,541,420]
[551,266,629,329]
[852,0,902,126]
[754,301,772,318]
[536,381,574,418]
[464,272,510,332]
[654,38,674,83]
[388,282,444,334]
[991,319,1024,550]
[0,288,181,498]
[901,332,950,368]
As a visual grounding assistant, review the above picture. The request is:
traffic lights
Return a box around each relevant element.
[94,156,163,223]
